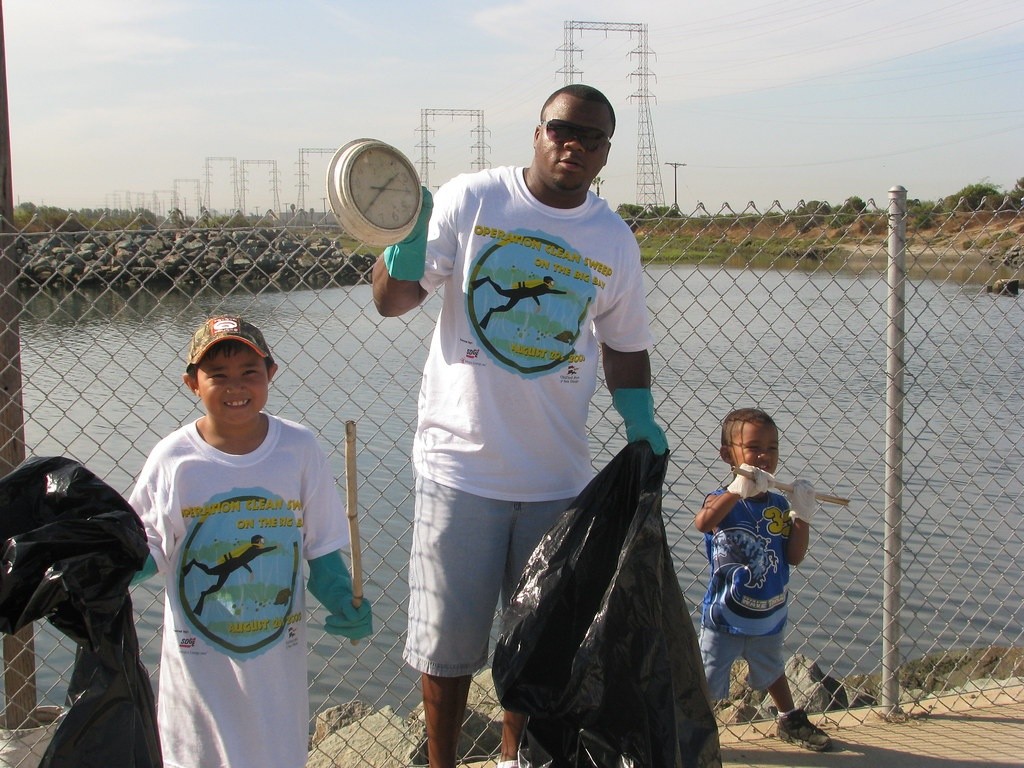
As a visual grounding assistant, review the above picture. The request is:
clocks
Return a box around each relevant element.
[349,146,420,230]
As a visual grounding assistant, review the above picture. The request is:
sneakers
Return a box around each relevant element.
[776,708,832,750]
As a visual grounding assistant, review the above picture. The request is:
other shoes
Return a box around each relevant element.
[496,759,518,768]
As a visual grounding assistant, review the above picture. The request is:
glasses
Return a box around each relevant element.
[541,119,611,151]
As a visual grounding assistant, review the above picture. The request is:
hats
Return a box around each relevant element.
[185,315,274,367]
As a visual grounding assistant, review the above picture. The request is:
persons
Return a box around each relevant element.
[699,408,833,751]
[129,314,373,768]
[372,83,670,768]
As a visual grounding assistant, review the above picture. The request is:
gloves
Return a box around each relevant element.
[382,186,434,281]
[788,479,815,524]
[612,387,669,455]
[727,462,774,499]
[307,549,373,639]
